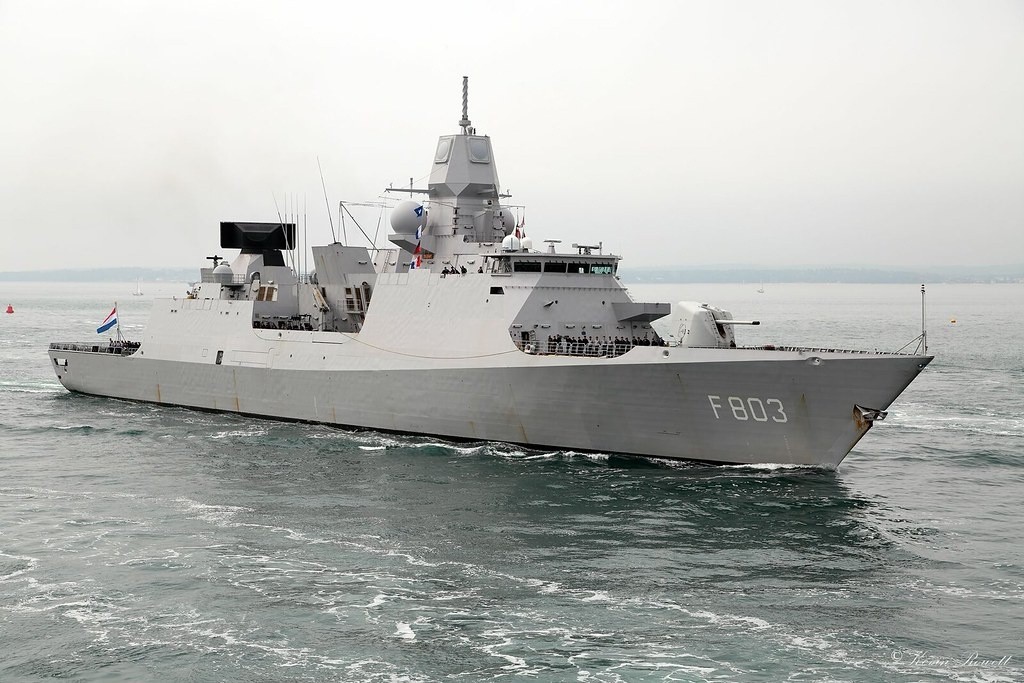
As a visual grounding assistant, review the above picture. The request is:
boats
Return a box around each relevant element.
[46,74,937,474]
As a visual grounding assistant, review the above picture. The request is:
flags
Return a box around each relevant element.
[410,255,420,269]
[96,307,117,334]
[415,224,422,239]
[413,205,423,218]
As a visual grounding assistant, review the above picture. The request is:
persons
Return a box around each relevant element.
[547,333,664,358]
[109,340,141,355]
